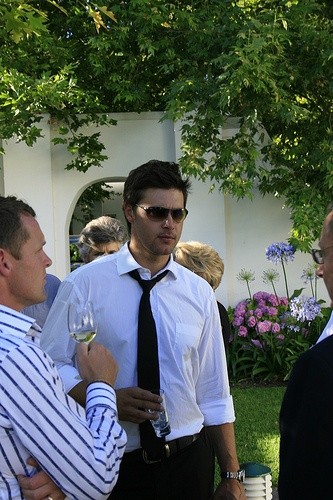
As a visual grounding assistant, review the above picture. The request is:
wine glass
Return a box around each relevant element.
[67,300,98,381]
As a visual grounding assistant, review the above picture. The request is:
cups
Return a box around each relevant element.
[144,389,172,438]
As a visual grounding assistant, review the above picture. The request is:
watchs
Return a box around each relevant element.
[219,469,245,483]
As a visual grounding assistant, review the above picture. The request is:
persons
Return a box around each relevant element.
[277,202,333,500]
[18,214,232,392]
[0,193,128,500]
[39,158,246,500]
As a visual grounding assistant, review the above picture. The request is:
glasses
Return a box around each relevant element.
[134,202,189,226]
[311,245,333,265]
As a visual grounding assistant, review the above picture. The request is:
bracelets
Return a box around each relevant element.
[85,378,118,392]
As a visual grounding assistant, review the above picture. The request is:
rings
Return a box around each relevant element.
[47,495,53,500]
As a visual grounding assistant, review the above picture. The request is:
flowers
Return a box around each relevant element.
[224,241,326,383]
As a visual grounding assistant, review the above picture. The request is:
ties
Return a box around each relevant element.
[126,270,171,454]
